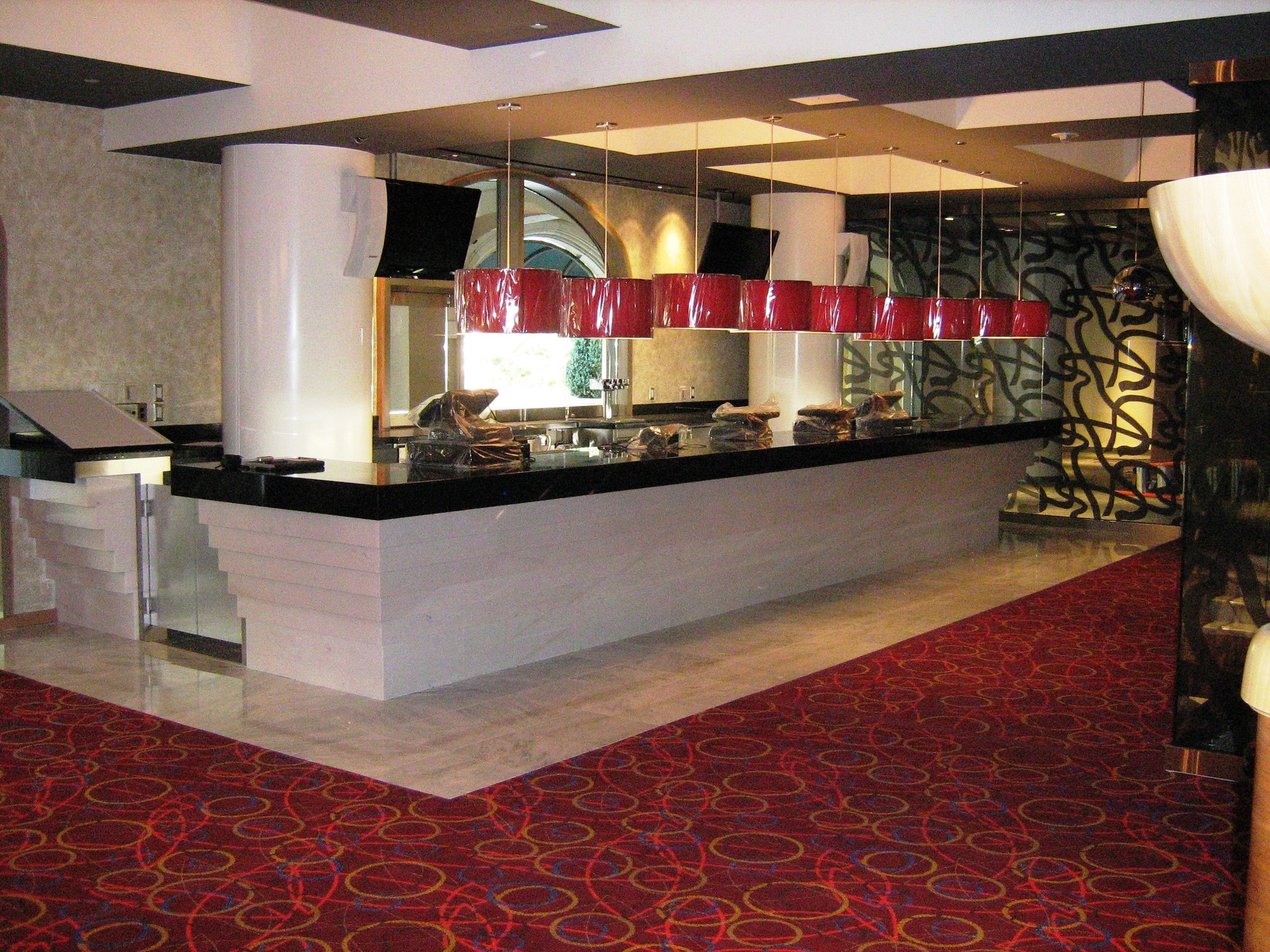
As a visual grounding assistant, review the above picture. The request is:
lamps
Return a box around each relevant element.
[1110,79,1159,309]
[447,102,1056,345]
